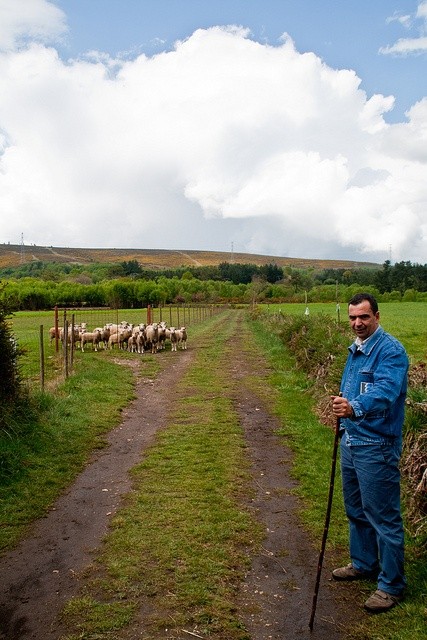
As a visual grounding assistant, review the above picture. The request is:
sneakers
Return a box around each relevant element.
[332,562,369,580]
[364,589,404,612]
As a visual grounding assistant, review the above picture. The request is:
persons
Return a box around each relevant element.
[330,293,410,614]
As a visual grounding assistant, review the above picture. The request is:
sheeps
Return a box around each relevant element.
[109,320,167,354]
[81,329,104,352]
[58,323,82,351]
[68,322,87,331]
[93,322,112,350]
[72,329,86,349]
[164,326,178,352]
[175,325,188,350]
[49,327,62,350]
